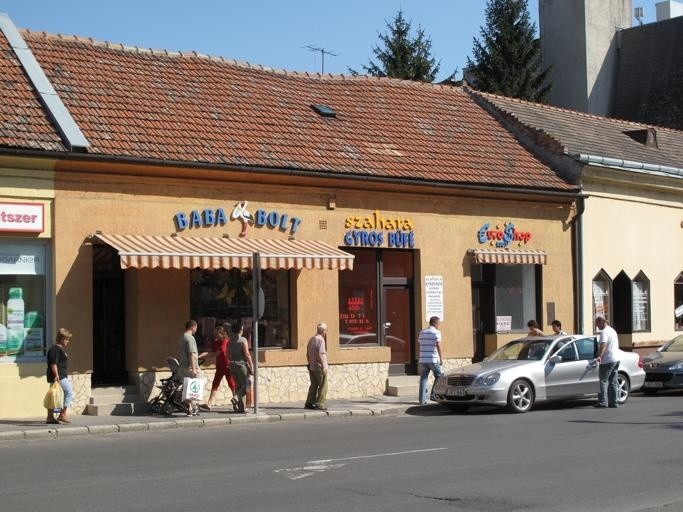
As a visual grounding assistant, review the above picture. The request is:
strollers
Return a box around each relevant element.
[150,354,207,417]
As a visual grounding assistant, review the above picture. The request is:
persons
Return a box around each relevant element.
[443,315,445,405]
[592,317,621,408]
[227,327,253,414]
[526,320,544,358]
[198,327,237,411]
[47,328,72,423]
[177,321,198,416]
[551,321,567,336]
[304,323,329,410]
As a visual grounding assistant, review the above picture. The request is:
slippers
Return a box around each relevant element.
[46,416,72,424]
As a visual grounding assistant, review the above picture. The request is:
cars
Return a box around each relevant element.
[338,332,408,357]
[640,334,682,391]
[432,333,646,414]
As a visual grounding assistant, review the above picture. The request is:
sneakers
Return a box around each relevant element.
[594,403,617,408]
[305,404,327,410]
[188,404,210,417]
[231,398,249,412]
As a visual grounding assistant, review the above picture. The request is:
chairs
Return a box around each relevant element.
[564,348,572,361]
[533,349,544,360]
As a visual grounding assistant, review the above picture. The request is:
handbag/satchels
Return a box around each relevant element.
[181,377,204,402]
[246,375,254,408]
